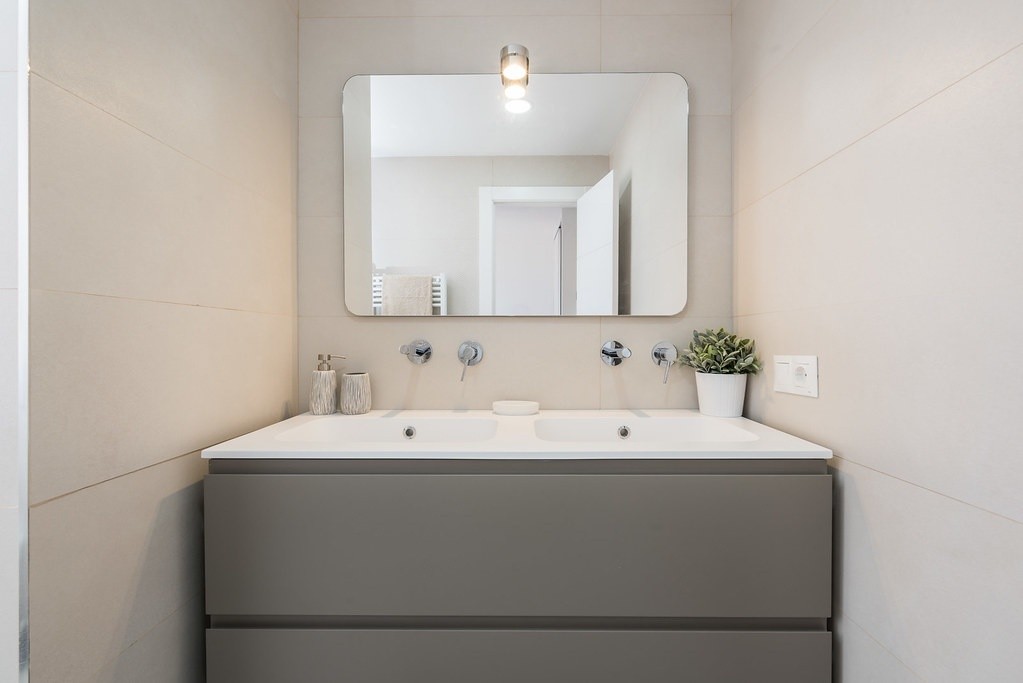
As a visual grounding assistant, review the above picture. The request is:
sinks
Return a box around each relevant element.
[273,415,499,443]
[533,415,760,443]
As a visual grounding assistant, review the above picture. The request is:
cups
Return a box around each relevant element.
[340,372,371,415]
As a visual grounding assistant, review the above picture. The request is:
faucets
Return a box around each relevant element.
[400,339,433,364]
[600,340,632,367]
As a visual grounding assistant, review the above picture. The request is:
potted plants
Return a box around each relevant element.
[677,328,759,418]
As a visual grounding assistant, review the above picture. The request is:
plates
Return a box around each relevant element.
[492,401,539,415]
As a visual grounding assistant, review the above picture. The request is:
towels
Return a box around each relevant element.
[382,273,433,315]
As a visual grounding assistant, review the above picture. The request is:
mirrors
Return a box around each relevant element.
[342,72,688,317]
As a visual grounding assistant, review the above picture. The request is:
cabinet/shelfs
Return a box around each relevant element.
[201,467,839,683]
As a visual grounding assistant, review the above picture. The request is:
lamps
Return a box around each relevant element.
[498,43,530,98]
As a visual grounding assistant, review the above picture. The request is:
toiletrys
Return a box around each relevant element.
[310,354,346,416]
